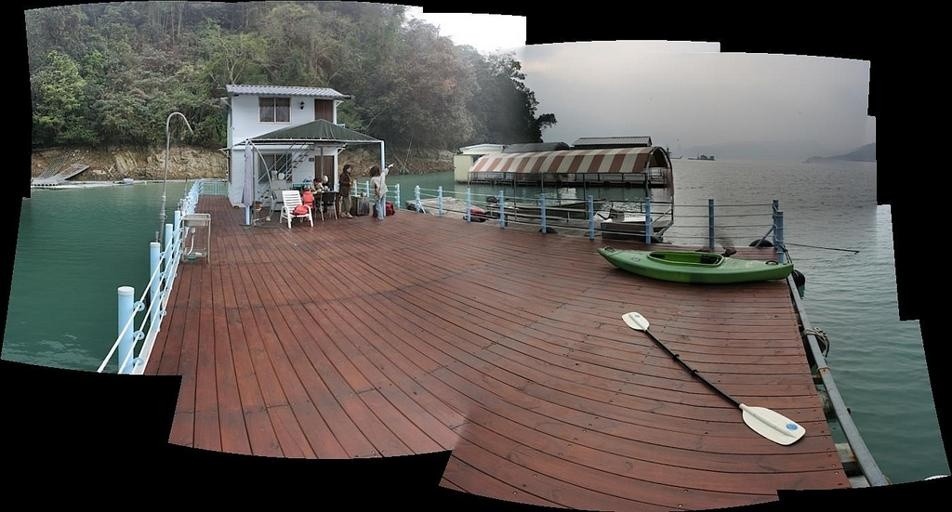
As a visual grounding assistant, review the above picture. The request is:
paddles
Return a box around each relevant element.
[622,311,806,446]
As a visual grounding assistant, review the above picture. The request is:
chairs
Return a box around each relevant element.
[279,184,338,229]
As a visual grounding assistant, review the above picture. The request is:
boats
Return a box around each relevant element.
[403,136,674,244]
[669,155,715,161]
[455,197,457,200]
[597,247,793,282]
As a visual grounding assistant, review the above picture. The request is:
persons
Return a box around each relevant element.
[370,163,394,220]
[300,175,332,210]
[339,165,354,219]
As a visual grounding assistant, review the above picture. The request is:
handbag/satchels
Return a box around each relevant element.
[386,201,394,215]
[294,205,307,214]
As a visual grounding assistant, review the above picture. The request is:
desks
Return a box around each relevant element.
[301,191,341,220]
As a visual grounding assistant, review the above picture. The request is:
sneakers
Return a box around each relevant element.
[341,212,352,218]
